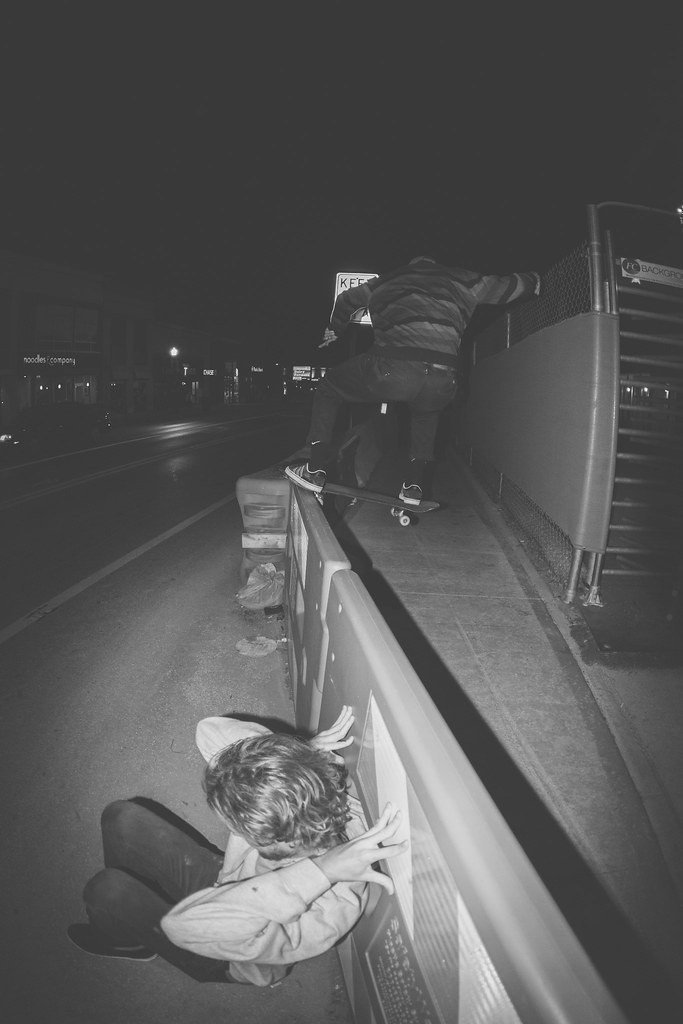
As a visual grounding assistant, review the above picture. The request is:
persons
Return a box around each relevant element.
[284,244,541,508]
[67,704,412,987]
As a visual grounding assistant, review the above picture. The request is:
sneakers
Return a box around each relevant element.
[67,923,158,961]
[399,482,424,505]
[285,462,327,493]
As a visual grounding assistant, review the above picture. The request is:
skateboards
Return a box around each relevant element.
[276,462,441,527]
